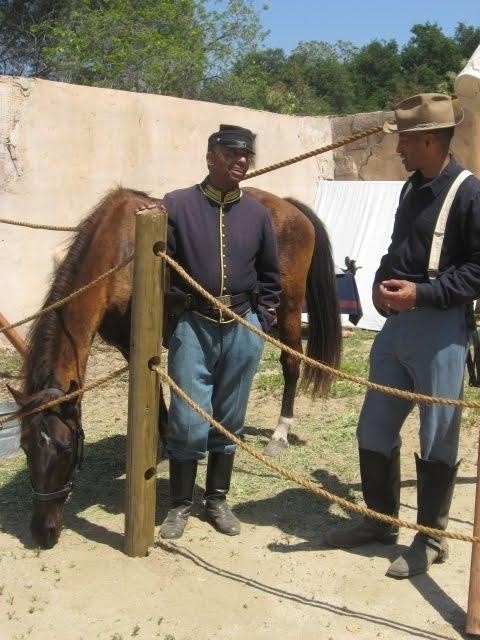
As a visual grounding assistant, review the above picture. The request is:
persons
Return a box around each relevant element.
[325,90,480,578]
[133,129,284,540]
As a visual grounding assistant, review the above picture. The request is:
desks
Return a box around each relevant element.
[301,269,364,327]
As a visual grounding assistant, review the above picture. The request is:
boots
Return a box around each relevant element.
[324,444,404,550]
[160,457,198,540]
[386,451,464,579]
[204,449,242,537]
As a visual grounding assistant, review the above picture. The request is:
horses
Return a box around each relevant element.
[5,186,343,550]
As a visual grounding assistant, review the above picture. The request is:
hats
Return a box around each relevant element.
[207,129,256,157]
[382,92,465,135]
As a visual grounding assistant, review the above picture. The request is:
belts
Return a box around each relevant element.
[192,292,250,311]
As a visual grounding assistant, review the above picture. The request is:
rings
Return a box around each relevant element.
[387,303,391,308]
